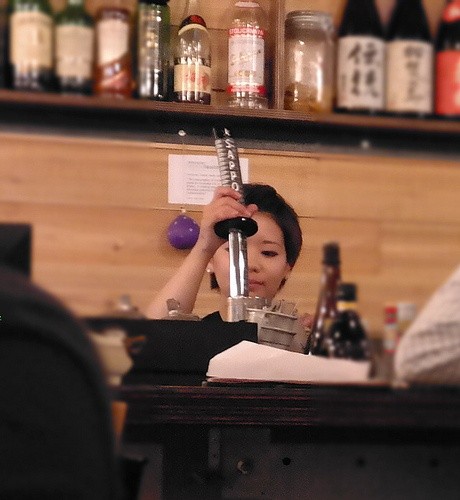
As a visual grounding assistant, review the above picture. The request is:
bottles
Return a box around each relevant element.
[93,0,135,99]
[375,307,403,380]
[284,9,334,114]
[5,0,52,92]
[173,14,211,105]
[51,0,95,95]
[384,0,435,118]
[134,0,173,102]
[305,242,344,358]
[334,0,385,116]
[433,0,460,121]
[326,282,366,361]
[225,0,270,111]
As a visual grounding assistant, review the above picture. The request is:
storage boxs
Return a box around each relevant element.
[124,318,260,375]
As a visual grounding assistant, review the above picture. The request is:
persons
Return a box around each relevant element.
[145,182,302,320]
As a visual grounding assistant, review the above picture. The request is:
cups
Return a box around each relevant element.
[246,307,298,351]
[225,297,266,322]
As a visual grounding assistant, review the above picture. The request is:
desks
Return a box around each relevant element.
[108,385,460,500]
